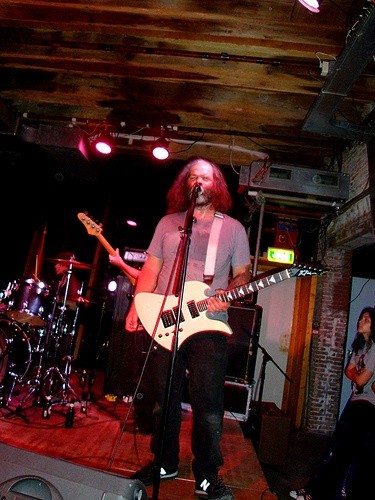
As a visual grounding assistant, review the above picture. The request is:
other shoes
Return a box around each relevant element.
[122,422,149,433]
[289,488,311,500]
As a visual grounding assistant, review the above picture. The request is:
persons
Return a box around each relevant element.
[109,247,157,433]
[126,160,250,499]
[51,252,79,309]
[289,307,375,500]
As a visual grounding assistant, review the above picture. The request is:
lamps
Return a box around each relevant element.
[152,138,170,161]
[94,133,114,155]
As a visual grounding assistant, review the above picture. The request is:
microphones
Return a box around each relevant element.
[191,182,204,195]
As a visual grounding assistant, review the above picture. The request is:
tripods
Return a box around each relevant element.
[0,263,87,425]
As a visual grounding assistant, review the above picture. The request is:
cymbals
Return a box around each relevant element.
[54,296,95,305]
[47,257,98,270]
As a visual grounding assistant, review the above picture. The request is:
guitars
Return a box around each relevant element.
[133,264,332,352]
[78,212,138,286]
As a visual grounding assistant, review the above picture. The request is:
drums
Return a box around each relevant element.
[6,277,55,326]
[0,302,8,319]
[0,316,34,385]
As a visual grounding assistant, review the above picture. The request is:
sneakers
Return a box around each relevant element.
[194,476,234,500]
[130,462,178,486]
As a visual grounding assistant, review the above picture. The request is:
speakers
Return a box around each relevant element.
[0,442,148,500]
[225,292,263,384]
[239,165,250,185]
[180,377,253,422]
[274,220,318,263]
